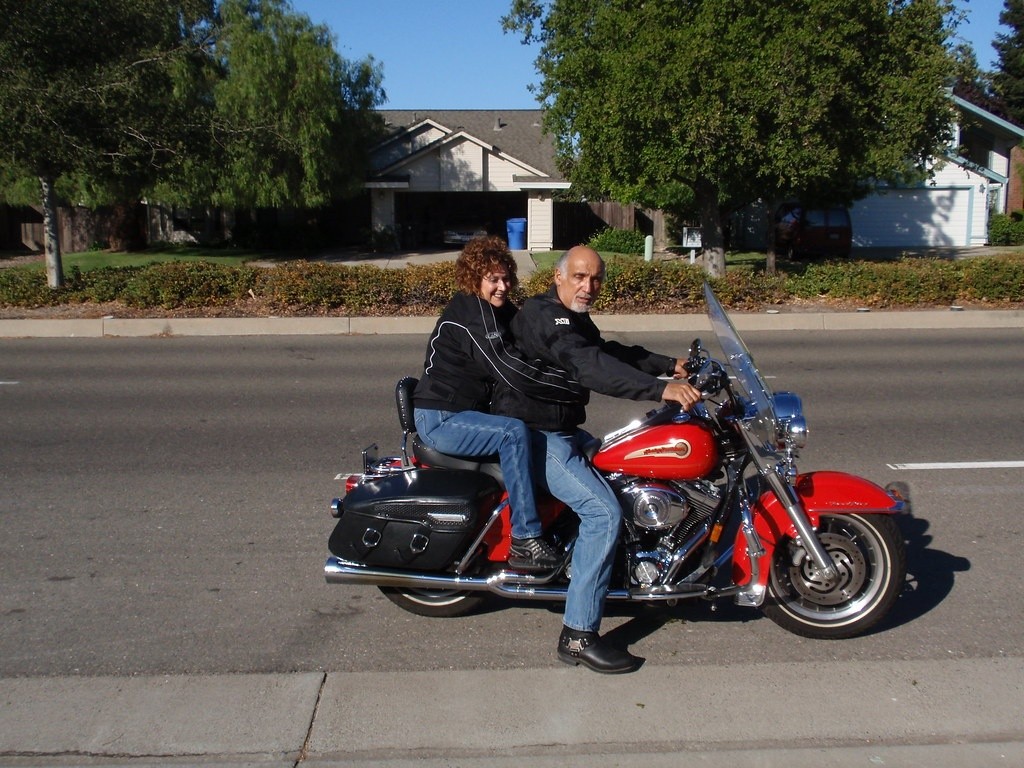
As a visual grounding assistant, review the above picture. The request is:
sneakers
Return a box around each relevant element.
[507,535,563,569]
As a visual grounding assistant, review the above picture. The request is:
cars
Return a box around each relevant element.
[442,208,494,250]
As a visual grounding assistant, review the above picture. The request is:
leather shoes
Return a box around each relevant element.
[557,624,638,673]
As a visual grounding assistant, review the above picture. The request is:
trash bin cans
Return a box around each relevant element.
[507,218,527,249]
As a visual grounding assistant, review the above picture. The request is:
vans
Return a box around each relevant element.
[764,201,853,262]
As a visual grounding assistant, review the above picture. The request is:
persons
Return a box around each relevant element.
[488,247,704,677]
[415,235,569,569]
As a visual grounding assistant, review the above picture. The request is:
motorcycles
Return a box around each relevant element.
[322,281,908,641]
